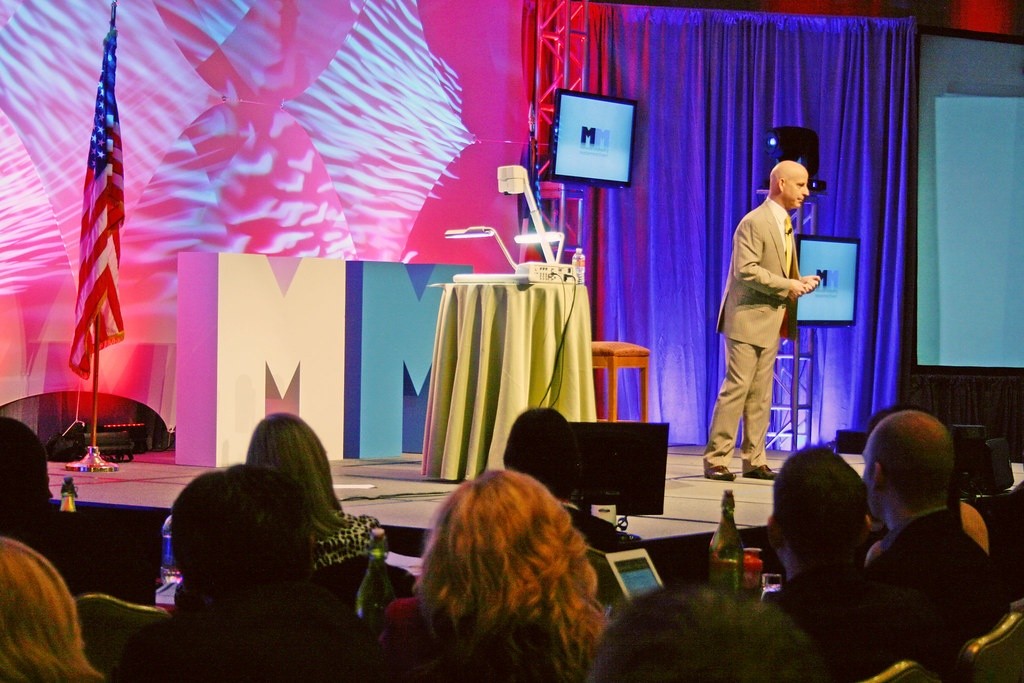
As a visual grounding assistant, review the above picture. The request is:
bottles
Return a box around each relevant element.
[59,477,78,512]
[354,526,398,642]
[709,488,744,601]
[572,248,586,285]
[760,572,781,597]
[159,514,182,585]
[742,547,764,590]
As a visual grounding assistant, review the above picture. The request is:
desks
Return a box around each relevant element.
[441,284,587,484]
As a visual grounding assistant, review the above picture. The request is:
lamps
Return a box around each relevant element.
[444,226,565,283]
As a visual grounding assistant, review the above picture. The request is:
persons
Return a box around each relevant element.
[859,401,1024,683]
[503,408,620,555]
[704,160,821,481]
[247,413,417,641]
[0,535,105,683]
[605,588,828,683]
[111,466,371,683]
[763,448,960,683]
[373,470,605,683]
[0,415,54,553]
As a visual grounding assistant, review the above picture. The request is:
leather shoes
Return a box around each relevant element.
[743,464,779,480]
[704,466,736,481]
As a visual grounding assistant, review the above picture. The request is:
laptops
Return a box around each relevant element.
[606,547,667,596]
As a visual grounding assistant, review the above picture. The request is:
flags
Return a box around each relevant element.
[67,6,127,379]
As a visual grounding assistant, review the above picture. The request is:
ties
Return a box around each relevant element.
[785,216,792,279]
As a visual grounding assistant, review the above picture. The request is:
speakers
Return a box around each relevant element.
[949,425,1014,495]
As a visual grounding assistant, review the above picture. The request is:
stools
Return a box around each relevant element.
[592,340,652,423]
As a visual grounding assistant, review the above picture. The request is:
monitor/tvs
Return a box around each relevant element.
[570,422,670,516]
[793,233,861,328]
[548,88,637,188]
